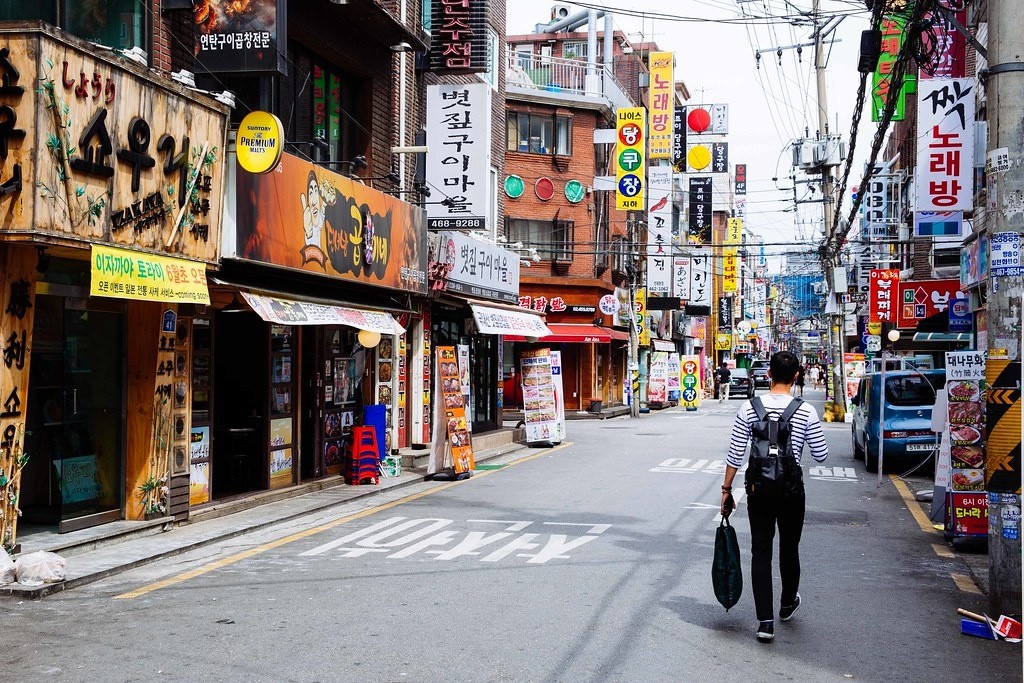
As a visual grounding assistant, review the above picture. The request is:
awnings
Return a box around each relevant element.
[468,297,554,338]
[504,323,611,344]
[237,289,407,335]
[602,326,630,340]
[286,0,432,53]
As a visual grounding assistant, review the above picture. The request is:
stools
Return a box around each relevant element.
[343,424,381,486]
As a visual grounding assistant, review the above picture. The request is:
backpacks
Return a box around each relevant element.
[744,396,805,500]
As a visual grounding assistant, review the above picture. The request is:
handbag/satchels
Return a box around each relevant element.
[711,514,743,612]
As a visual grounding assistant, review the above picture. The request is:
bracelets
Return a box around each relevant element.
[721,485,732,494]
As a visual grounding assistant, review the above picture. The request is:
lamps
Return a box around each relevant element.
[592,317,605,324]
[357,330,382,348]
[492,235,543,268]
[384,186,431,197]
[185,86,237,110]
[352,174,401,185]
[87,40,149,68]
[410,198,455,208]
[284,137,330,151]
[887,329,901,342]
[311,156,369,170]
[525,336,539,344]
[152,64,196,88]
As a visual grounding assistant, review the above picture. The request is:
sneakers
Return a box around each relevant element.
[779,592,802,620]
[757,621,775,641]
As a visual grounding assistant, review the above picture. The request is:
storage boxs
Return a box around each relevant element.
[843,412,854,424]
[381,455,402,477]
[995,614,1022,638]
[364,403,387,460]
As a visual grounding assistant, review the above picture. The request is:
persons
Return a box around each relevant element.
[720,352,829,640]
[766,368,772,388]
[792,360,827,398]
[718,362,733,404]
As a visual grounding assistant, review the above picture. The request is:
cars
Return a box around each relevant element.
[850,367,947,475]
[751,360,771,368]
[750,367,772,390]
[714,368,756,399]
